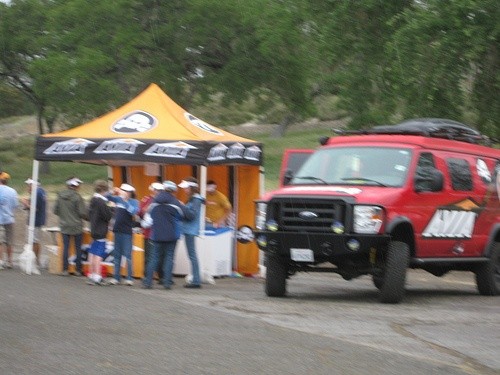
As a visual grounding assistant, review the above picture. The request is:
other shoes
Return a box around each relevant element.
[141,285,151,289]
[184,284,202,289]
[109,278,120,286]
[74,271,82,276]
[0,261,14,270]
[62,271,69,276]
[125,280,134,286]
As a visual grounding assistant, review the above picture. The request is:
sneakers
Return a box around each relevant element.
[85,275,107,287]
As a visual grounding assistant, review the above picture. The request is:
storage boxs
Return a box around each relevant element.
[45,245,59,273]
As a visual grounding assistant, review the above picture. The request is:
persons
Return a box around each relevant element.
[0,171,232,290]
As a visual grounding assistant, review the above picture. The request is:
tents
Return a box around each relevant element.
[24,81,265,284]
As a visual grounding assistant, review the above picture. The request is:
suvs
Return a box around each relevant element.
[249,118,500,304]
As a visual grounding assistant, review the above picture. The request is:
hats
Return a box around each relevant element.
[151,180,166,190]
[206,184,217,193]
[65,177,84,187]
[24,179,41,185]
[0,172,11,181]
[178,179,199,189]
[120,183,136,192]
[163,181,178,190]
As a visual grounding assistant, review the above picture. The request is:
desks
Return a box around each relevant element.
[44,226,232,278]
